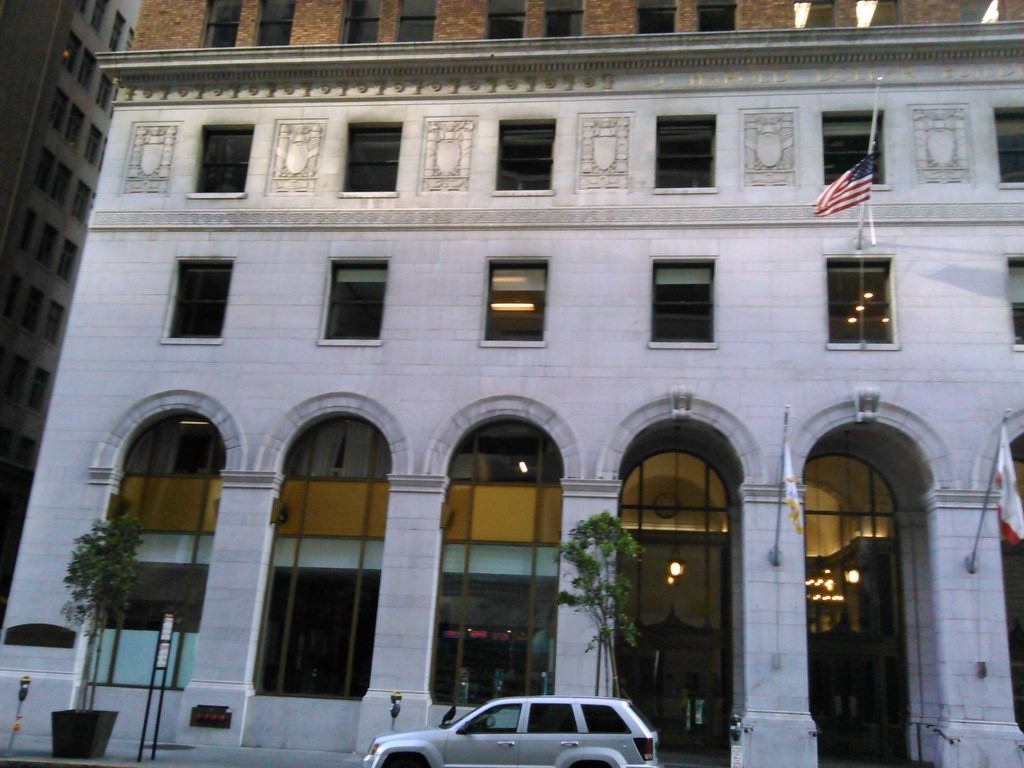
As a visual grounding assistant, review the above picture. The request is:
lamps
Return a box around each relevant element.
[841,430,864,586]
[666,425,686,578]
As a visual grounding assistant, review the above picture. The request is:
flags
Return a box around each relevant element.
[783,430,804,535]
[813,153,875,218]
[995,421,1024,548]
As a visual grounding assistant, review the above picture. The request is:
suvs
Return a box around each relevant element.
[360,694,664,768]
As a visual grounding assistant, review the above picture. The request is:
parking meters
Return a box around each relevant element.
[387,690,402,731]
[728,713,742,768]
[3,676,33,757]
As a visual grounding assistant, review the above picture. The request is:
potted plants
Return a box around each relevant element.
[49,510,146,754]
